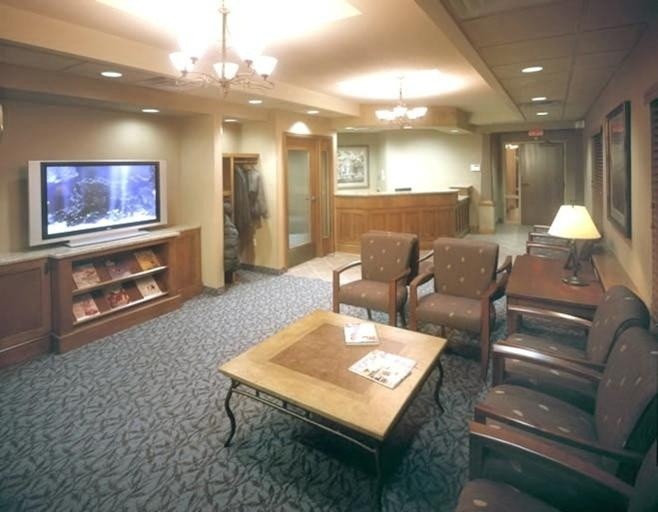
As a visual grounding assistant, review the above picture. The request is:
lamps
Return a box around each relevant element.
[374,75,427,130]
[548,203,600,286]
[169,0,276,99]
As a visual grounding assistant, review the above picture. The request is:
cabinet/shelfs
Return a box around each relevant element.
[0,226,201,370]
[222,151,260,285]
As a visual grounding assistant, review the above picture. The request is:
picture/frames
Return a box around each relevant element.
[605,100,630,244]
[335,144,369,191]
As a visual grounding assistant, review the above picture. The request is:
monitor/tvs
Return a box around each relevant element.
[29,160,168,248]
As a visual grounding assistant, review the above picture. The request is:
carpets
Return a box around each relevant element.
[0,269,585,512]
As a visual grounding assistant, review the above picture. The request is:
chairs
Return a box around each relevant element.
[332,232,421,327]
[410,237,511,377]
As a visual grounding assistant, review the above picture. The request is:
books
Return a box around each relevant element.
[348,350,418,390]
[344,322,379,347]
[71,248,162,319]
